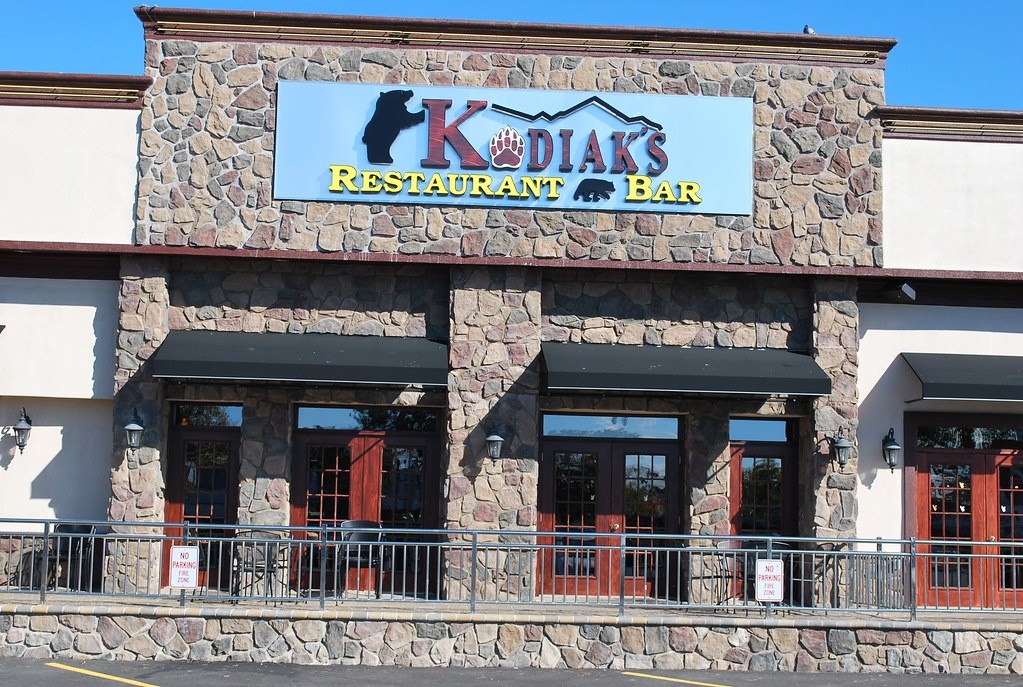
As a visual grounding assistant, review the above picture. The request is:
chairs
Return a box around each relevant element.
[737,542,794,617]
[187,529,226,603]
[336,520,392,601]
[40,523,98,593]
[234,529,289,609]
[797,543,847,609]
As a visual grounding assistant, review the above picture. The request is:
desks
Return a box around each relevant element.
[689,551,850,613]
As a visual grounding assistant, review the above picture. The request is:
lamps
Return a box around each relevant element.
[124,409,144,448]
[829,425,852,475]
[13,407,32,453]
[882,428,902,475]
[485,422,505,467]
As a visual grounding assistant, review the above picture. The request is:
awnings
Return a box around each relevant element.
[901,352,1023,403]
[152,329,449,384]
[541,341,833,396]
